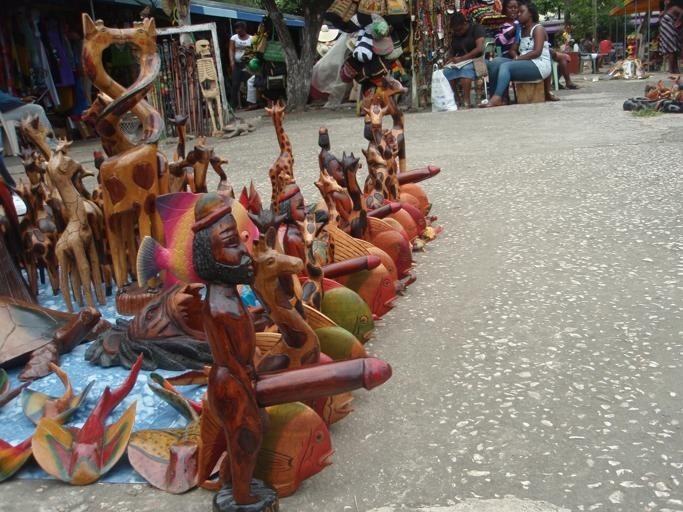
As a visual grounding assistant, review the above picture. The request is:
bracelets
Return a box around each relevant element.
[515,55,517,60]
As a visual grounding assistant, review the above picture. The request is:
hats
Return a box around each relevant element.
[318,25,339,42]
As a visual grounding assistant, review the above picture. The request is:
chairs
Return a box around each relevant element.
[443,37,494,106]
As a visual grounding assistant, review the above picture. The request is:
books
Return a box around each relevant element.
[442,58,473,70]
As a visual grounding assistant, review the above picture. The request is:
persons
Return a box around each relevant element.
[657,0,683,75]
[187,91,441,511]
[441,11,485,112]
[498,1,560,102]
[546,47,579,90]
[227,20,254,110]
[477,2,549,109]
[559,21,623,75]
[241,74,259,111]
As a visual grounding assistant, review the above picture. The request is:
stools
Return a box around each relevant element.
[512,79,545,103]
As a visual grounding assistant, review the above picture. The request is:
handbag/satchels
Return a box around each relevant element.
[340,12,408,84]
[263,26,286,93]
